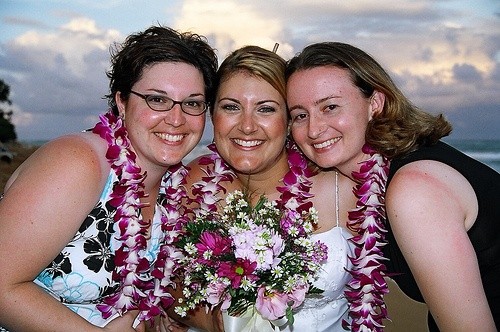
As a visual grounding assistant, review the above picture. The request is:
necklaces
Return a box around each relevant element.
[92,110,190,321]
[344,154,391,332]
[183,139,323,327]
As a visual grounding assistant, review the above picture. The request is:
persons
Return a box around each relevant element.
[286,42,500,332]
[179,46,363,332]
[0,26,217,332]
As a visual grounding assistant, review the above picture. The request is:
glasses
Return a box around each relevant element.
[130,90,210,116]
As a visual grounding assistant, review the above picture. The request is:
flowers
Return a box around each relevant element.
[170,186,330,330]
